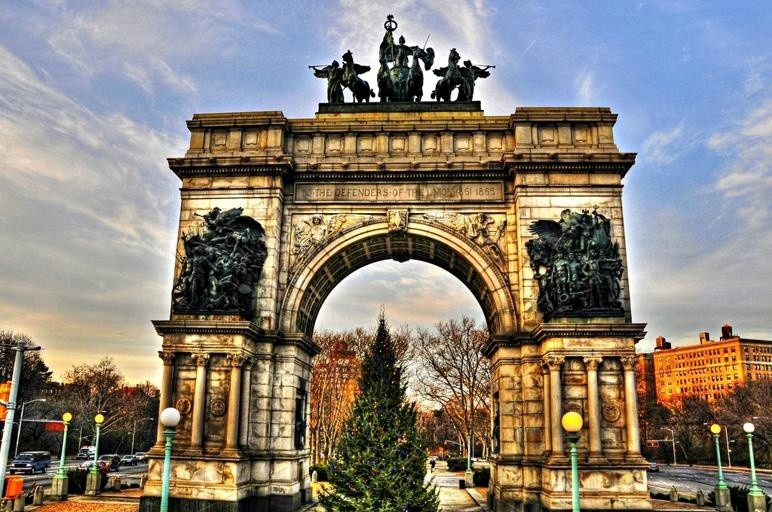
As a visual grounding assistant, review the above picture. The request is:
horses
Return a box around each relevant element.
[340,50,375,103]
[431,48,466,102]
[406,46,424,102]
[377,46,401,103]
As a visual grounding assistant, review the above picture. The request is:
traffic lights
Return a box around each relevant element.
[646,441,659,447]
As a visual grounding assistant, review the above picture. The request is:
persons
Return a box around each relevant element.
[473,211,494,245]
[525,207,624,316]
[307,213,331,241]
[460,58,489,101]
[177,206,267,317]
[311,57,350,102]
[392,34,413,65]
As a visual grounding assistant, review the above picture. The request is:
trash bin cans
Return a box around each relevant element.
[459,480,465,489]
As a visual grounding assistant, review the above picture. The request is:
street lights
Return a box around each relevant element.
[131,416,154,454]
[709,423,734,510]
[660,427,677,465]
[561,411,584,511]
[742,422,768,511]
[50,412,73,501]
[703,421,735,467]
[464,433,474,487]
[443,439,463,446]
[14,398,47,459]
[84,413,105,496]
[159,407,180,512]
[78,410,107,452]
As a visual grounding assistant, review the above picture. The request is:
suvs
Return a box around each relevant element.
[10,450,51,475]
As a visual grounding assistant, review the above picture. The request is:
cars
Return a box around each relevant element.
[646,462,659,472]
[76,445,149,474]
